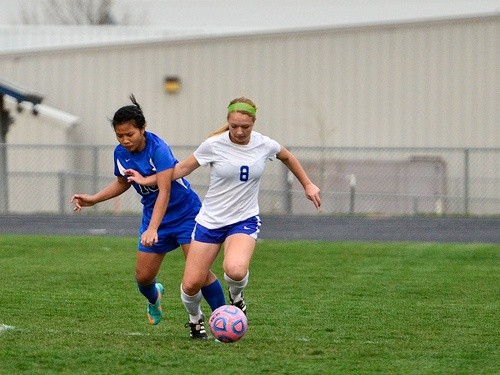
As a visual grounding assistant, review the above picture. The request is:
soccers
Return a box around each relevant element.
[208,305,248,343]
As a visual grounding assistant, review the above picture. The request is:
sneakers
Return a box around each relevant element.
[146,283,164,325]
[189,313,208,339]
[228,291,247,316]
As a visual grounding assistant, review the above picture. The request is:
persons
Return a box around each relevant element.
[123,96,321,341]
[70,93,226,325]
